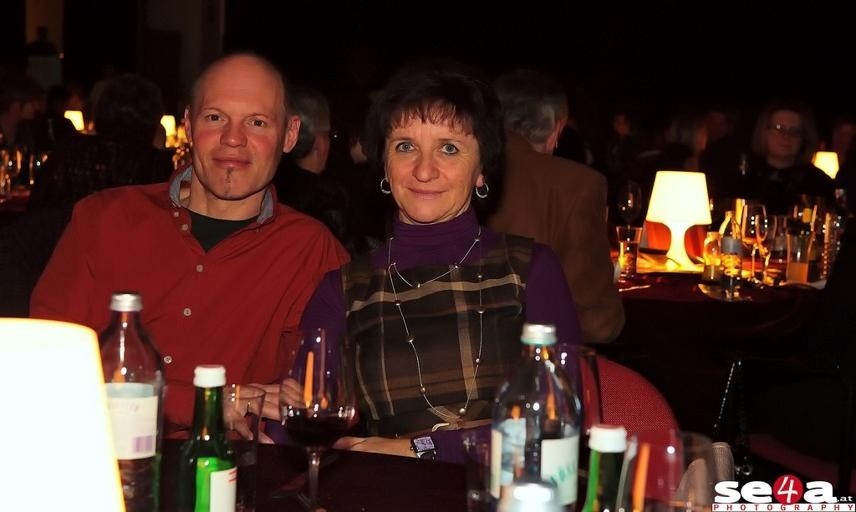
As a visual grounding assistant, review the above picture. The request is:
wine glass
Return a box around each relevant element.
[277,326,359,511]
[616,182,641,228]
[740,204,778,286]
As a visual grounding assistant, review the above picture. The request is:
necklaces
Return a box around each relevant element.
[387,227,485,419]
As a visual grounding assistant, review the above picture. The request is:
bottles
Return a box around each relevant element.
[97,290,167,511]
[484,322,584,511]
[702,231,721,282]
[174,364,238,512]
[718,210,742,276]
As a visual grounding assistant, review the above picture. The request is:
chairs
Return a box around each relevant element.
[579,356,685,503]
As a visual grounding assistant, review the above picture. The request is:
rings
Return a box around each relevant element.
[245,400,252,413]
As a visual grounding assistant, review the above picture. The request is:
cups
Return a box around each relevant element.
[615,226,644,278]
[221,382,268,469]
[785,226,815,283]
[613,429,719,511]
[461,428,491,511]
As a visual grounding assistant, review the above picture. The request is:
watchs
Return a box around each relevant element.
[410,433,437,461]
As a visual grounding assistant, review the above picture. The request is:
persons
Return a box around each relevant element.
[1,24,852,344]
[29,54,350,446]
[265,62,584,470]
